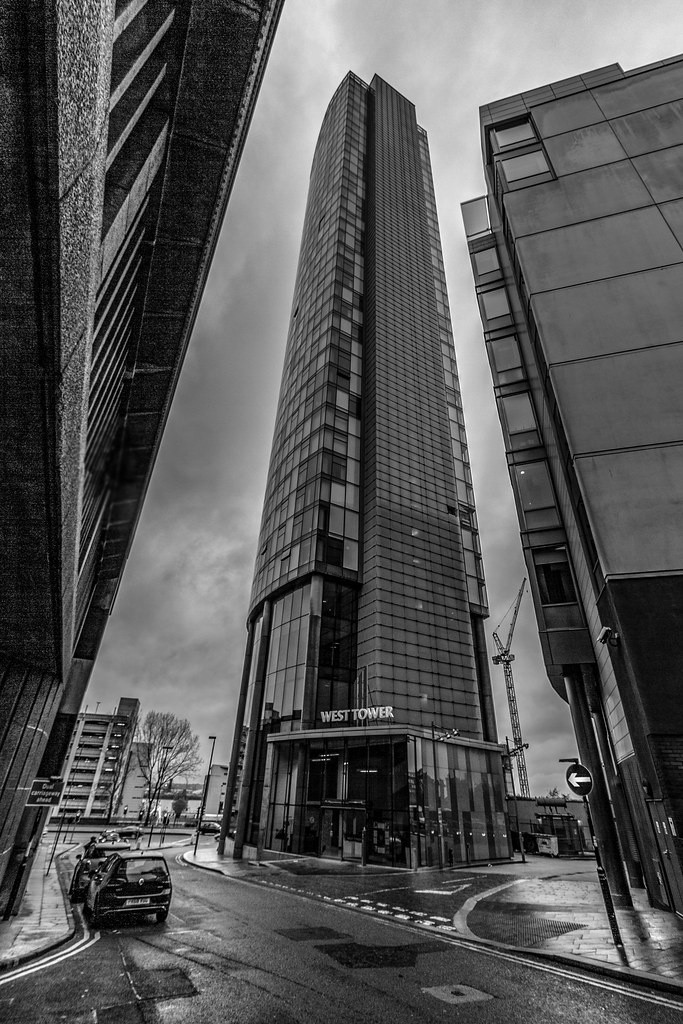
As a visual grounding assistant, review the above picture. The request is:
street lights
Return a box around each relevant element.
[194,736,216,854]
[148,746,174,846]
[559,758,621,943]
[508,743,529,862]
[432,729,459,869]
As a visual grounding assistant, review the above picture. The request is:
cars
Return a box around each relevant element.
[68,844,132,901]
[83,851,173,928]
[214,828,237,841]
[196,821,221,835]
[112,826,144,839]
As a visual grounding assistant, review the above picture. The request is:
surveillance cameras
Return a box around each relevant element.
[597,627,612,645]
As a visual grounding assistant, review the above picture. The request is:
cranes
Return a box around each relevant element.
[491,577,526,797]
